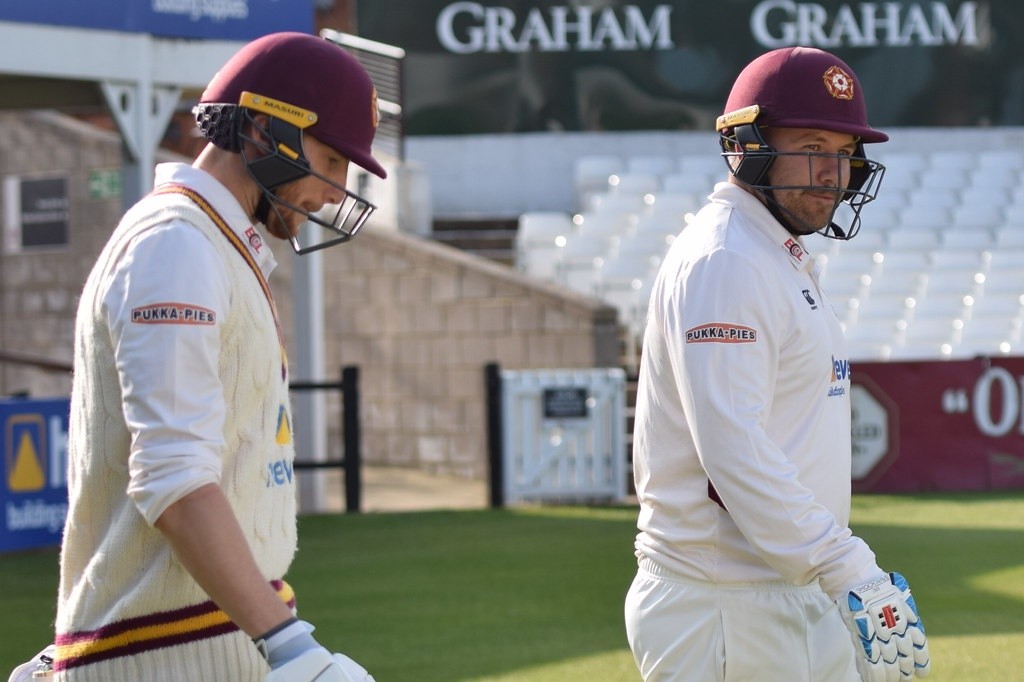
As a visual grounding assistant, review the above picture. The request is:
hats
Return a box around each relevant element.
[722,47,888,153]
[200,32,386,187]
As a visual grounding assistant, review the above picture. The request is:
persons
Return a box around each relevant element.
[621,46,933,682]
[5,30,384,682]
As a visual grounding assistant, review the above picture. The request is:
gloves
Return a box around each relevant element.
[835,566,928,681]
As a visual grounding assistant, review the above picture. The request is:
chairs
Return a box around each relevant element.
[514,151,1024,359]
